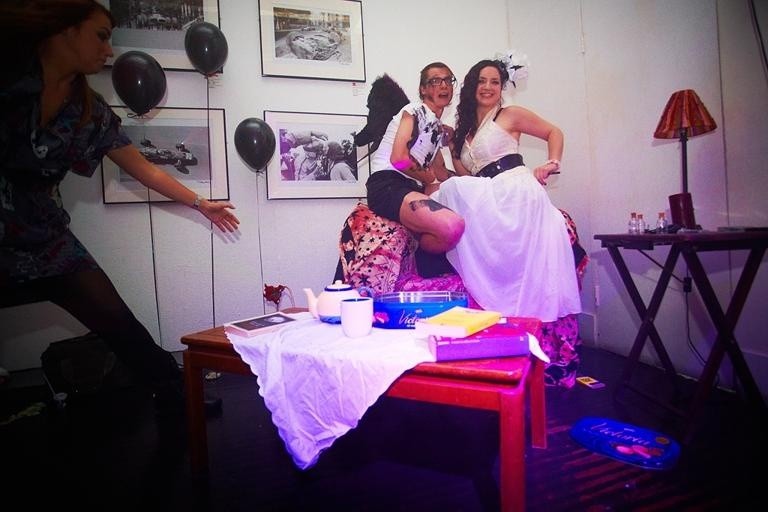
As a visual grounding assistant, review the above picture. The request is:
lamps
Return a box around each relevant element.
[654,89,718,230]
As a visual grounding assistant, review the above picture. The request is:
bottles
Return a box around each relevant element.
[637,214,645,234]
[656,212,669,235]
[628,212,639,234]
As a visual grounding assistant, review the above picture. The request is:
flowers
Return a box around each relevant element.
[263,281,297,311]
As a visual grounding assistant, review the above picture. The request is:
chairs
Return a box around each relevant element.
[332,199,590,391]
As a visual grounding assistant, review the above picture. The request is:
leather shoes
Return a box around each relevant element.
[151,378,223,413]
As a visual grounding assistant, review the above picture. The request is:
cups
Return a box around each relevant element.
[53,392,68,411]
[339,296,373,339]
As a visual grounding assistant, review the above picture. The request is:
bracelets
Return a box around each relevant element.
[191,194,203,209]
[546,159,561,169]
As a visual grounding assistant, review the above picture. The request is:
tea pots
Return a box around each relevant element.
[302,280,372,325]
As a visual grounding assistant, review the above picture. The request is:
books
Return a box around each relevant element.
[413,305,528,362]
[226,311,297,339]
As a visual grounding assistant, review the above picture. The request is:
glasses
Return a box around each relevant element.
[426,76,456,86]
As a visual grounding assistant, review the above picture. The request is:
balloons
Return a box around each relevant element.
[234,118,276,174]
[184,22,228,76]
[111,50,166,116]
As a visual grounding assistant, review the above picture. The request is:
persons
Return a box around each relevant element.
[426,59,583,323]
[365,62,465,279]
[1,3,240,415]
[280,129,357,181]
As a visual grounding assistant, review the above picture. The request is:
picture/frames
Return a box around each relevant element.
[100,104,230,205]
[258,0,367,82]
[264,108,371,201]
[93,0,223,74]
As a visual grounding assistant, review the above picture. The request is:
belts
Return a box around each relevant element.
[474,153,525,178]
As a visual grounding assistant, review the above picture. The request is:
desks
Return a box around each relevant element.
[593,224,768,419]
[181,307,548,512]
